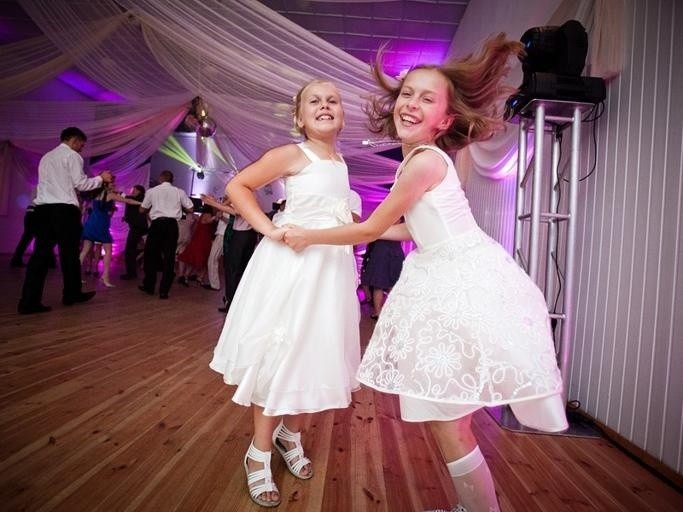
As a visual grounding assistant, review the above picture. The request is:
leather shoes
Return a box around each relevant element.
[79,269,232,313]
[16,304,52,315]
[61,290,97,306]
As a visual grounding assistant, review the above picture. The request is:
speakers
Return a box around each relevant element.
[516,18,588,81]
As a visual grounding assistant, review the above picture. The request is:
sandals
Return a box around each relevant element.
[271,415,314,479]
[242,435,282,507]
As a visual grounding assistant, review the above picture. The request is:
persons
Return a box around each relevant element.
[284,29,571,512]
[15,125,112,314]
[221,77,363,509]
[349,189,406,321]
